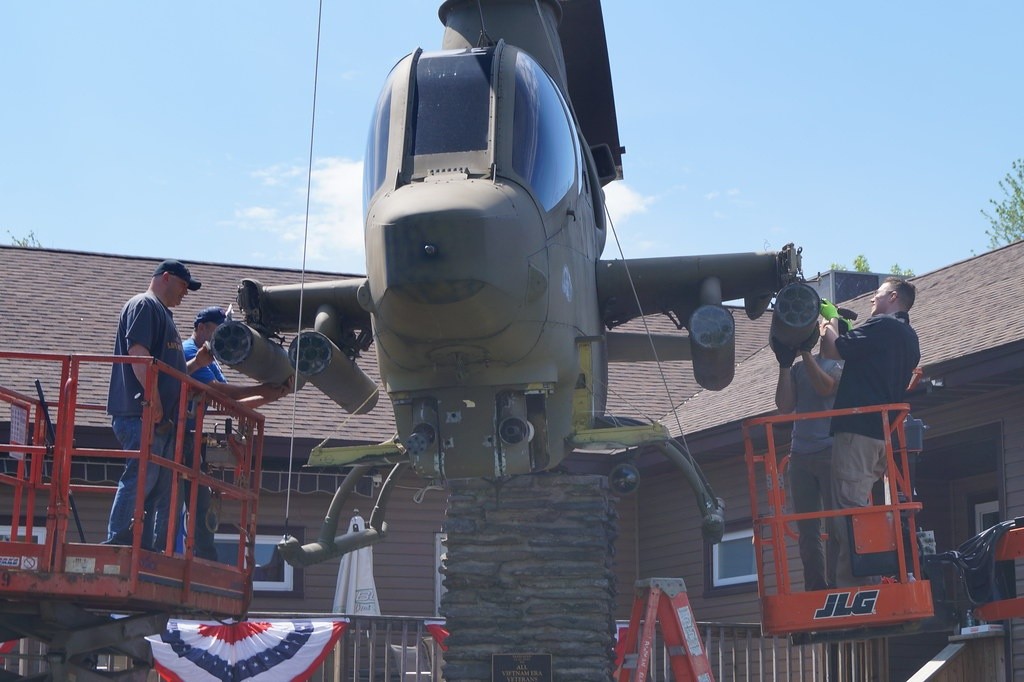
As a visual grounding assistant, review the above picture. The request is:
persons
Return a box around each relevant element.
[768,305,844,592]
[181,306,295,561]
[824,278,922,587]
[106,260,202,552]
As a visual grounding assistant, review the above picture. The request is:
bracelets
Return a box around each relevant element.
[780,364,790,368]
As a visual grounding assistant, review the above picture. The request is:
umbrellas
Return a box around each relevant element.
[330,516,382,615]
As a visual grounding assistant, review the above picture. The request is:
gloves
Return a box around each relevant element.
[840,318,853,331]
[801,341,812,353]
[780,347,797,369]
[819,298,839,321]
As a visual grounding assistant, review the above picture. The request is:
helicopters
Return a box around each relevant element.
[210,0,821,571]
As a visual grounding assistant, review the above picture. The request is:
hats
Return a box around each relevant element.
[195,306,226,326]
[152,259,201,291]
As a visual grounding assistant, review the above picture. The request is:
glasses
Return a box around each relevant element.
[874,289,899,298]
